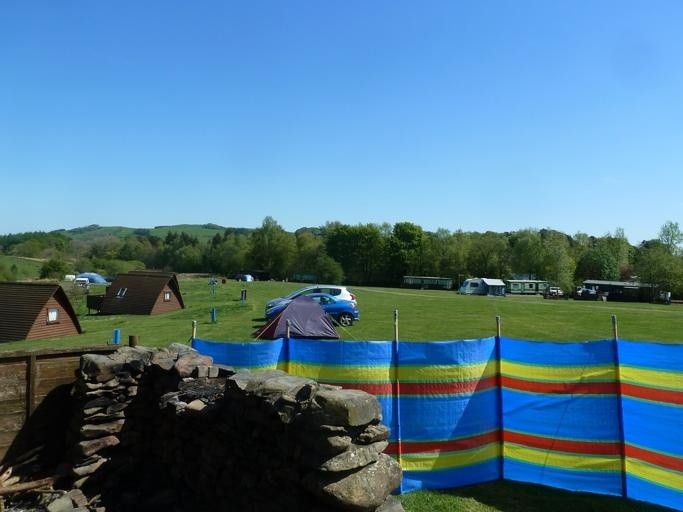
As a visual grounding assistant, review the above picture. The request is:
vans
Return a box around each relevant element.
[458,278,487,295]
[73,278,89,284]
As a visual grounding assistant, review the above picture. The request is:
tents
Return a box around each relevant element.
[248,294,341,338]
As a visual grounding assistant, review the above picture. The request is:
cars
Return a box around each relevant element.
[575,287,596,296]
[542,286,564,298]
[264,284,360,327]
